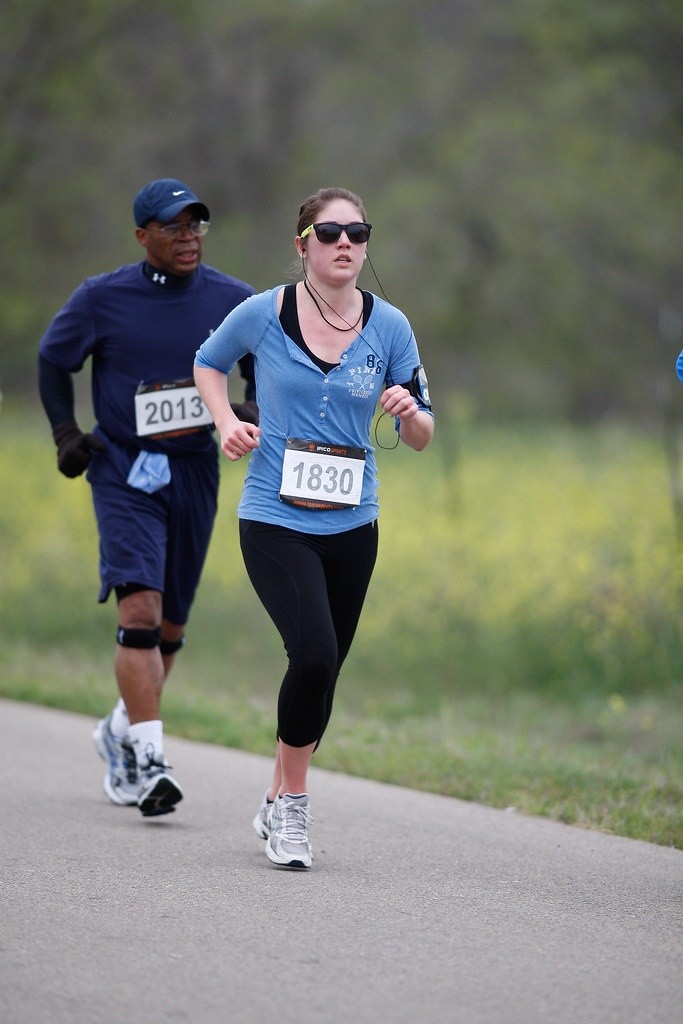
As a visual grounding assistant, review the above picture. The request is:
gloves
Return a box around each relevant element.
[52,420,95,477]
[230,401,260,428]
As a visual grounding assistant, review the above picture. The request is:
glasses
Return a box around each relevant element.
[144,222,209,237]
[300,222,373,245]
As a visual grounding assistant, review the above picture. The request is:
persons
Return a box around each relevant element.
[37,179,265,820]
[190,188,437,871]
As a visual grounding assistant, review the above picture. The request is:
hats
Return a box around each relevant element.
[133,179,211,230]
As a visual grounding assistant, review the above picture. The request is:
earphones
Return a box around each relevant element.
[300,245,305,252]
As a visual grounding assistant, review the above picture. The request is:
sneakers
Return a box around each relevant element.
[265,790,313,869]
[93,712,139,805]
[254,788,273,839]
[136,764,183,816]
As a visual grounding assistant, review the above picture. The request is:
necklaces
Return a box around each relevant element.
[304,279,365,332]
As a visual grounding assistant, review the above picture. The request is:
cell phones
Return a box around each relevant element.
[413,364,432,407]
